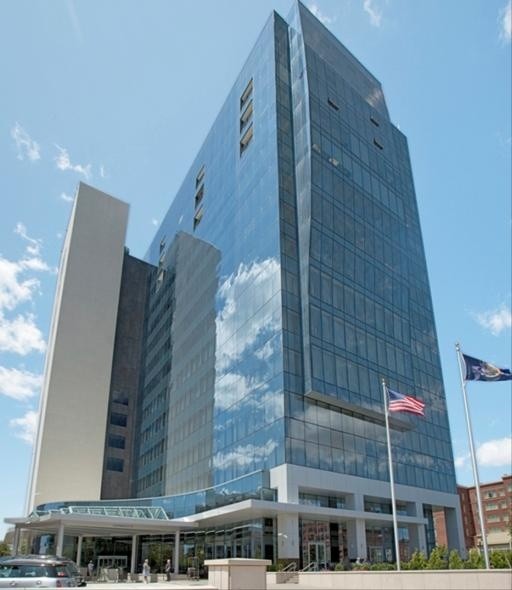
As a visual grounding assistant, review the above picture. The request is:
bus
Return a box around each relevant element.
[84,555,128,575]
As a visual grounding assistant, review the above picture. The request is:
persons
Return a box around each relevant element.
[165,558,171,581]
[86,557,95,577]
[141,558,151,583]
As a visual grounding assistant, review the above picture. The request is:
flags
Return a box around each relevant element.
[385,386,427,417]
[462,352,512,383]
